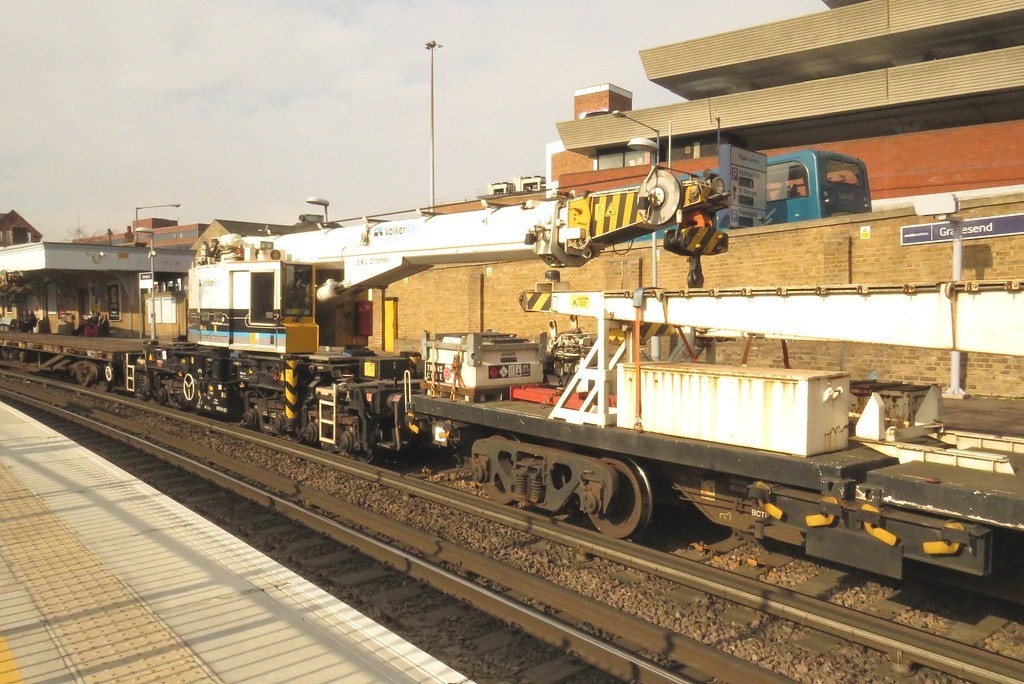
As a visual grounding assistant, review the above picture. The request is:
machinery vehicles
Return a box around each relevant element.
[180,170,731,349]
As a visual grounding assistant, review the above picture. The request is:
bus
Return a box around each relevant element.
[596,148,873,243]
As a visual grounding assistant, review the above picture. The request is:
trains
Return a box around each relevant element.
[0,164,1024,591]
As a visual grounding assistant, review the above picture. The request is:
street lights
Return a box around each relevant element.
[306,197,331,228]
[424,40,442,210]
[134,202,181,244]
[617,109,662,165]
[136,226,158,342]
[625,140,661,290]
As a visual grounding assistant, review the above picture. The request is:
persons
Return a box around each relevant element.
[21,310,36,332]
[60,309,110,338]
